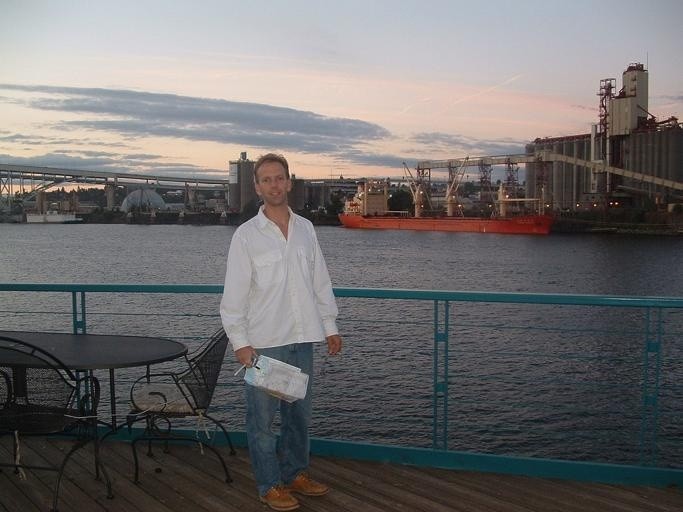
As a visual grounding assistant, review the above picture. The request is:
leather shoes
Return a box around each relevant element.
[284,471,331,497]
[259,485,300,512]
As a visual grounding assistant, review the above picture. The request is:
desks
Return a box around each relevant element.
[0,328,188,481]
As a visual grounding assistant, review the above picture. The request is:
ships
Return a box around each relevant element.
[335,152,558,236]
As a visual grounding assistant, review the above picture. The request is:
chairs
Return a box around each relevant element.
[0,332,116,511]
[123,325,239,488]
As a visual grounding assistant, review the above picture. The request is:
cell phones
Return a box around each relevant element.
[252,355,260,370]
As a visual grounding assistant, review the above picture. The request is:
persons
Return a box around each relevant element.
[218,153,344,510]
